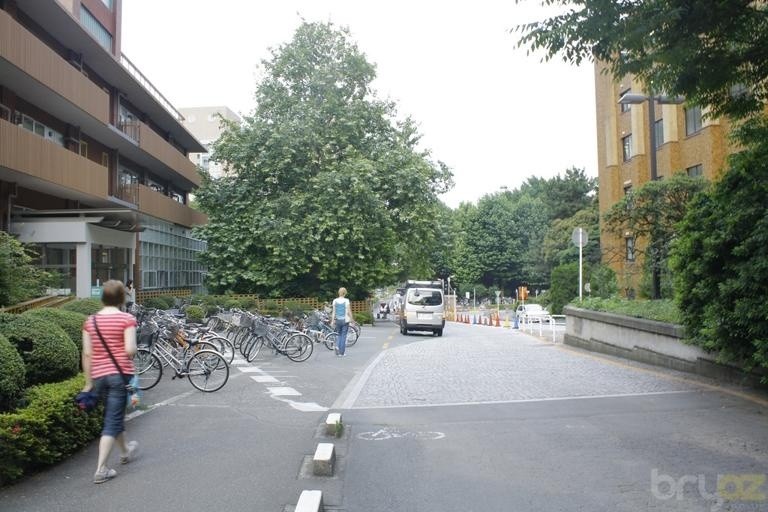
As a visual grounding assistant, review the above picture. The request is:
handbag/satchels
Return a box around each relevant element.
[126,375,140,407]
[345,309,351,322]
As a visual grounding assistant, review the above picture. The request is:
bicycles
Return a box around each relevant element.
[127,297,358,394]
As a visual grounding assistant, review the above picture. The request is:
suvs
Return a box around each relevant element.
[515,304,551,323]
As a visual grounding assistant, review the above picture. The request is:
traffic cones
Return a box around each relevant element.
[494,315,501,327]
[454,314,481,324]
[487,314,493,326]
[502,313,510,327]
[482,318,487,325]
[511,314,518,329]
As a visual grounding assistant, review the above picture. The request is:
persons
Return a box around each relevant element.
[125,280,135,313]
[332,287,355,356]
[83,280,140,483]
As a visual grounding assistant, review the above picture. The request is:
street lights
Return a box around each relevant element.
[617,92,685,299]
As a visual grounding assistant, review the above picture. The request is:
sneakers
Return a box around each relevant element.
[93,469,116,483]
[336,348,346,357]
[121,440,138,463]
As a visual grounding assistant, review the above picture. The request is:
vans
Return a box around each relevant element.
[395,279,446,338]
[379,304,389,313]
